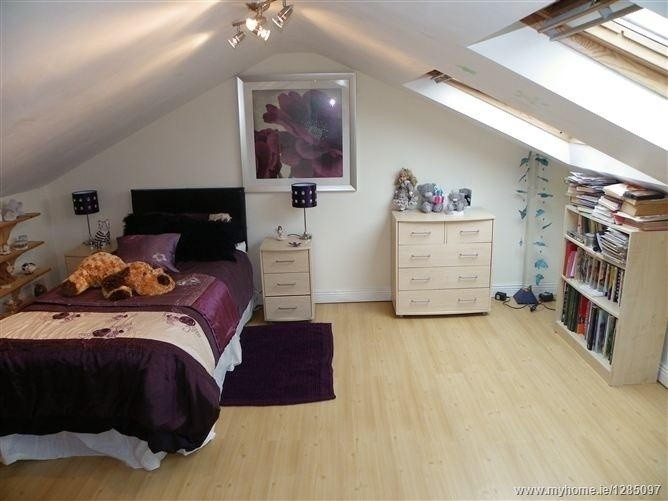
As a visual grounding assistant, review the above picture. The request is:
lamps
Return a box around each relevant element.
[71,190,99,246]
[227,1,294,48]
[291,183,317,241]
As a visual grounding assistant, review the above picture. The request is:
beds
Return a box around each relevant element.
[0,187,253,470]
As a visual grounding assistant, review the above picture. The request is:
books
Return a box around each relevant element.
[561,170,668,365]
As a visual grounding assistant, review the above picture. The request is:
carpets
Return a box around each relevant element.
[218,323,336,407]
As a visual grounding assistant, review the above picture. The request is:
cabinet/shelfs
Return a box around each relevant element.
[552,205,667,387]
[390,210,495,318]
[0,213,52,319]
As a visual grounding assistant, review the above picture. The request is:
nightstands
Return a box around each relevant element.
[65,239,119,275]
[259,235,316,323]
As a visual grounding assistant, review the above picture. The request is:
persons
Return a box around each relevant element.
[60,252,175,300]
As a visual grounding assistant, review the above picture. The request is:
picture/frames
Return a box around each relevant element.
[236,73,358,195]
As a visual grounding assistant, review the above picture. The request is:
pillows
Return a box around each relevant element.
[115,210,238,272]
